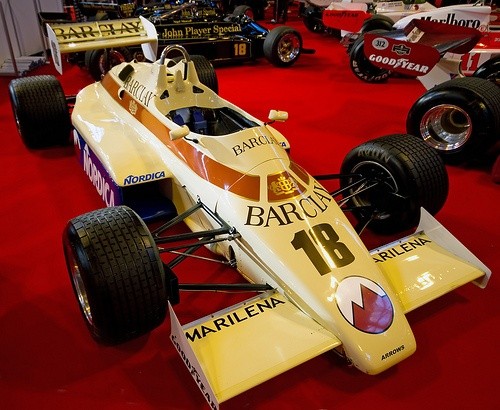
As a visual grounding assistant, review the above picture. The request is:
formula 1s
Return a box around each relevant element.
[8,0,500,410]
